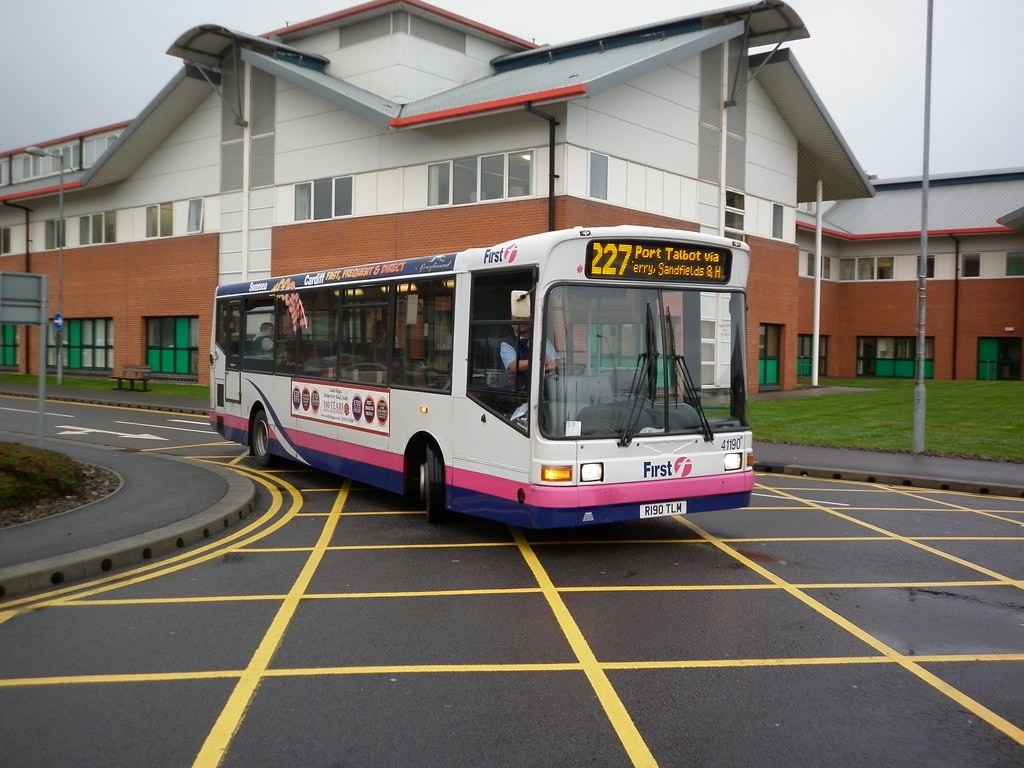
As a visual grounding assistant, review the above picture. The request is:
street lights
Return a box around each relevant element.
[26,147,67,385]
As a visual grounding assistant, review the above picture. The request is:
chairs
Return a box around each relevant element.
[339,354,364,365]
[341,363,387,383]
[398,357,433,388]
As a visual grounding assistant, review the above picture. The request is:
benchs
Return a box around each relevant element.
[110,365,153,393]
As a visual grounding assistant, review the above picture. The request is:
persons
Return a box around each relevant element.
[373,320,400,348]
[260,322,274,338]
[501,307,560,385]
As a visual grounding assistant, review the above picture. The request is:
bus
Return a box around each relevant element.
[208,224,755,524]
